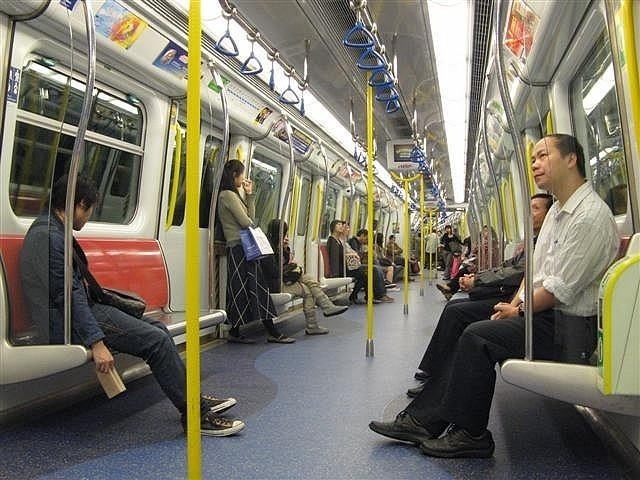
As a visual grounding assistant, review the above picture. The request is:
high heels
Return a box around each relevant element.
[350,294,383,304]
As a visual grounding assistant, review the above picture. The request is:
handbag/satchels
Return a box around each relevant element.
[101,287,145,319]
[283,263,302,286]
[240,227,274,261]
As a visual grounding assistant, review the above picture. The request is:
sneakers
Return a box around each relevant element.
[379,294,393,302]
[440,273,450,280]
[418,426,496,460]
[181,393,245,437]
[385,279,397,288]
[436,284,453,301]
[369,411,433,458]
[408,277,415,282]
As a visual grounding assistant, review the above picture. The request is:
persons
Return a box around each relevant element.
[17,171,246,437]
[368,133,623,461]
[324,217,525,304]
[217,158,296,344]
[405,192,559,399]
[259,217,350,335]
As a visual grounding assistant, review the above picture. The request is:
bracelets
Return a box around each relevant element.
[517,302,525,318]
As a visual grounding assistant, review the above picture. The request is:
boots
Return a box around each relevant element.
[305,305,329,335]
[317,295,348,317]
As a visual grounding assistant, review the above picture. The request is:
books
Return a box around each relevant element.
[90,361,128,401]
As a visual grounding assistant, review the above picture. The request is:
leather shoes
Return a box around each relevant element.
[267,333,296,344]
[407,372,430,398]
[227,332,256,344]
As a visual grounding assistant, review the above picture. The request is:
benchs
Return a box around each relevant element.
[0,234,229,387]
[500,234,640,424]
[317,246,354,289]
[271,281,328,307]
